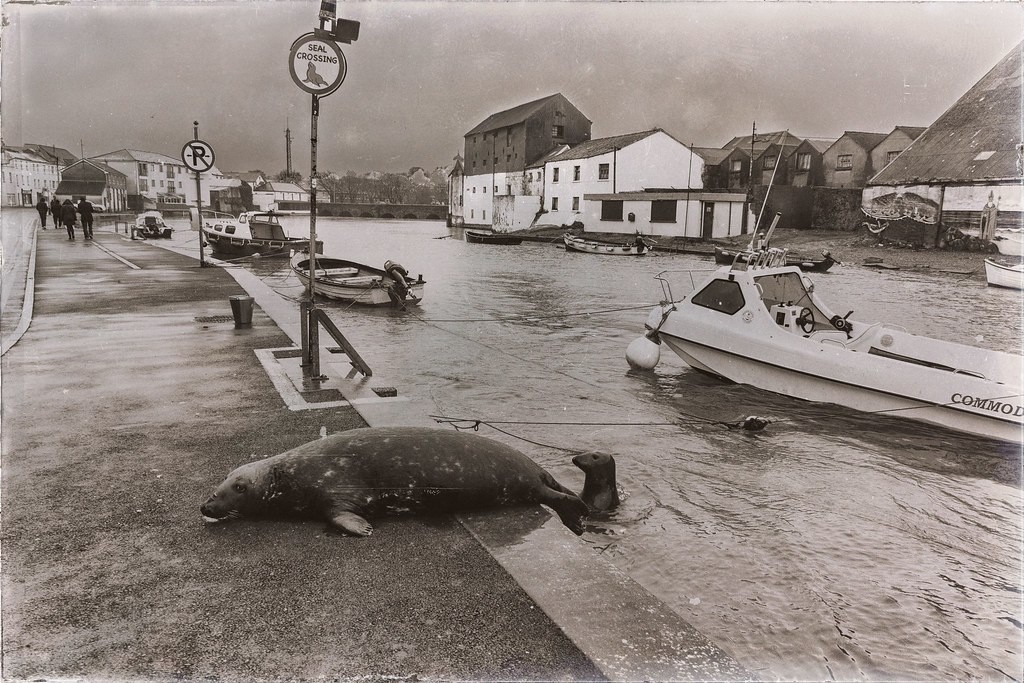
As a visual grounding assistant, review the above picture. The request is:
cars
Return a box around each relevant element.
[71,199,106,213]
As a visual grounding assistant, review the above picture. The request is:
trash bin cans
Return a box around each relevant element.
[228,295,256,323]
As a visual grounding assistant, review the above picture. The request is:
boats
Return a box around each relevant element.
[289,248,427,310]
[464,231,524,245]
[563,232,653,258]
[713,245,842,272]
[135,211,174,240]
[983,258,1024,290]
[624,213,1023,450]
[202,209,323,261]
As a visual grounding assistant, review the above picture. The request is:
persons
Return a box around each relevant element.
[630,236,650,250]
[78,197,94,240]
[61,198,77,239]
[35,196,62,229]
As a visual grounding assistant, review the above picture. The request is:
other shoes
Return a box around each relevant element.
[66,235,74,239]
[84,234,92,239]
[42,226,46,229]
